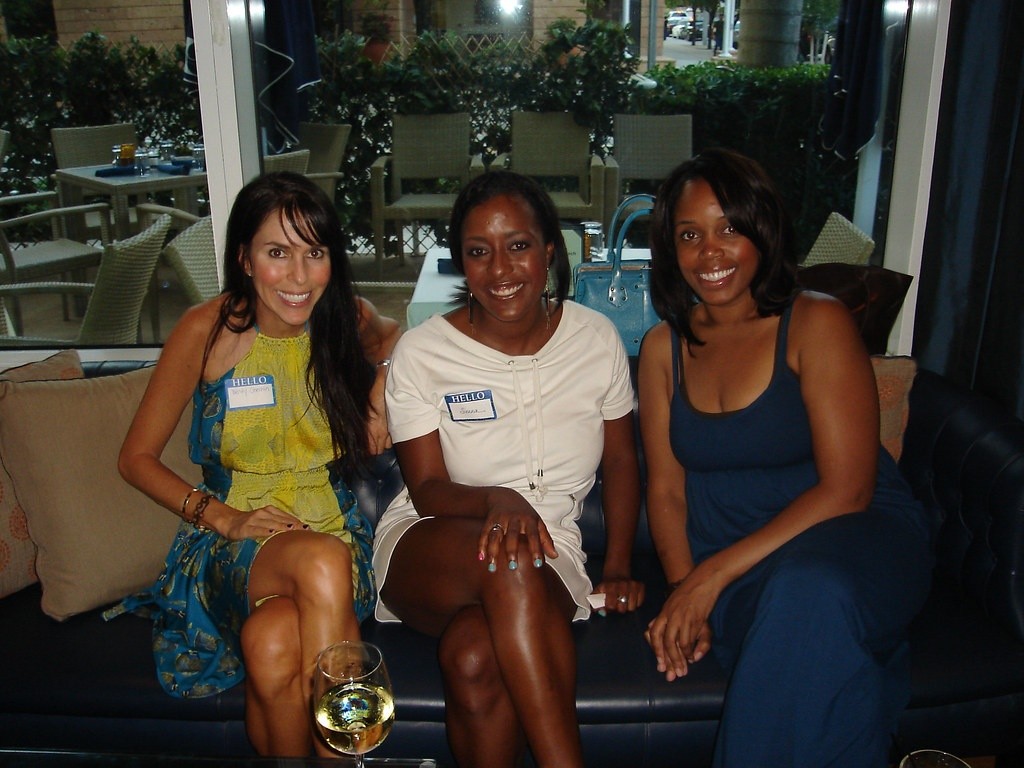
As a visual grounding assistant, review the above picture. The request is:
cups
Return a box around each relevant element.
[110,139,204,178]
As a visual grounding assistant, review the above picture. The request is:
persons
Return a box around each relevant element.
[118,173,402,759]
[369,169,644,768]
[636,147,937,768]
[663,14,808,65]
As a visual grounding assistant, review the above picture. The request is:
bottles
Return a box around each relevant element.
[580,220,605,263]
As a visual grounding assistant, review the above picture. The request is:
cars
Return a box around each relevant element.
[671,17,702,41]
[712,16,740,47]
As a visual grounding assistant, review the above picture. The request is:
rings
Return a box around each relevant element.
[490,522,505,534]
[617,594,628,603]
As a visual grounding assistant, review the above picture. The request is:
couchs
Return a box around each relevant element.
[0,364,1023,768]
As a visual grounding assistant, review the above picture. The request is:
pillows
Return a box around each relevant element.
[0,348,84,599]
[0,361,204,622]
[866,353,918,464]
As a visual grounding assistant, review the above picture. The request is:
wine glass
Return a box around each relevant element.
[312,640,397,768]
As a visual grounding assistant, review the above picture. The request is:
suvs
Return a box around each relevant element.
[667,11,688,25]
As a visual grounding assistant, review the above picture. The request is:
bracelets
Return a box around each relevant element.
[376,358,389,368]
[183,488,219,528]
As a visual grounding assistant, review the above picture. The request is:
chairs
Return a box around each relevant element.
[796,211,875,270]
[489,111,605,226]
[605,114,693,248]
[0,214,171,347]
[162,214,220,306]
[134,149,311,342]
[49,124,155,271]
[0,130,112,338]
[294,121,352,207]
[369,112,485,273]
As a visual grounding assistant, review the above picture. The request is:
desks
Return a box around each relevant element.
[406,248,651,331]
[54,162,208,317]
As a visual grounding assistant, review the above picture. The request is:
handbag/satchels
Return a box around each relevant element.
[785,260,914,355]
[573,191,660,358]
